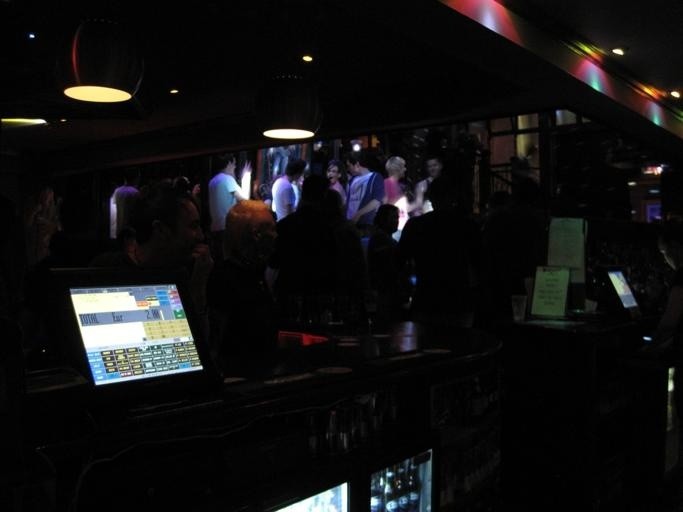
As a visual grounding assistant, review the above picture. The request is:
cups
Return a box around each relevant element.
[512,294,526,323]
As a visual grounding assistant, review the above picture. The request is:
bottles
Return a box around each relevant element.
[372,460,420,512]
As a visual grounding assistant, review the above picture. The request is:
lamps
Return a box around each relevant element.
[1,68,54,129]
[55,9,146,105]
[254,75,322,141]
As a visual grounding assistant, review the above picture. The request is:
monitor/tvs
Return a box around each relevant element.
[71,284,204,386]
[608,271,638,309]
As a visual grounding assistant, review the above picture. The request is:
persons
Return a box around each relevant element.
[99,183,214,332]
[110,143,632,363]
[641,220,683,433]
[26,187,56,259]
